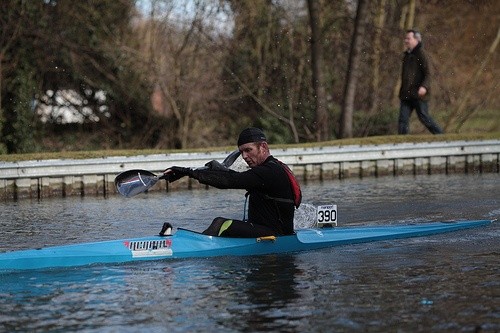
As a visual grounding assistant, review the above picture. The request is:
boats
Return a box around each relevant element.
[0,210,499,276]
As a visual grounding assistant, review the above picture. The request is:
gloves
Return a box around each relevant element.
[163,164,190,183]
[206,161,228,172]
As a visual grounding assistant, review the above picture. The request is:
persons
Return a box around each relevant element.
[397,30,445,134]
[163,128,302,237]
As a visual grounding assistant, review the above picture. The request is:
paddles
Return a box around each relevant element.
[114,149,240,198]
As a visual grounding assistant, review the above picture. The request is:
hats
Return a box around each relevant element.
[236,127,267,147]
